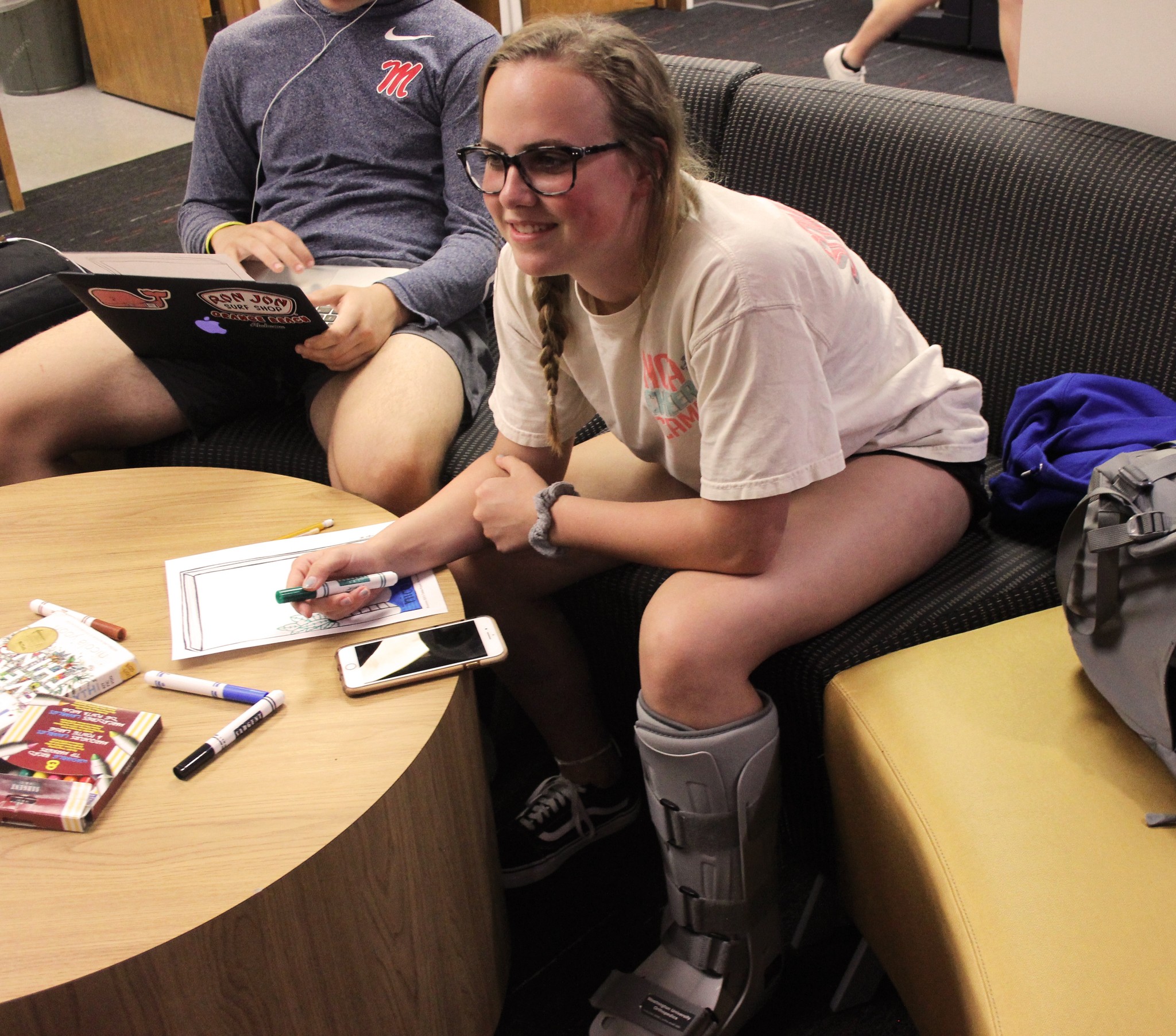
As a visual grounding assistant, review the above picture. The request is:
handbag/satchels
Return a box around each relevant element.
[1064,437,1176,833]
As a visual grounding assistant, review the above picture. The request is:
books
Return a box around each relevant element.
[0,610,141,736]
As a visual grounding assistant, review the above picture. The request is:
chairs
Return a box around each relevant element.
[448,55,1176,1036]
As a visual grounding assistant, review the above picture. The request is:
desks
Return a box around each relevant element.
[0,467,495,1036]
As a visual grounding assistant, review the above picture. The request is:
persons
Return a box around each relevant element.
[281,15,990,1036]
[0,0,505,518]
[822,0,1023,102]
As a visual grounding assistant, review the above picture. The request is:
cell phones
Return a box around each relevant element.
[336,615,508,697]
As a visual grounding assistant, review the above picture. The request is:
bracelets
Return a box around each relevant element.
[204,221,245,256]
[528,481,580,562]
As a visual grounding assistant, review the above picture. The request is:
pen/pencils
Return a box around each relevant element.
[282,518,335,539]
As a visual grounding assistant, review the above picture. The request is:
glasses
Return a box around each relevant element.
[455,139,625,197]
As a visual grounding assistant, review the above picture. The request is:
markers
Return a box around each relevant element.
[19,767,95,786]
[172,690,286,781]
[31,599,126,641]
[146,670,267,704]
[275,570,399,604]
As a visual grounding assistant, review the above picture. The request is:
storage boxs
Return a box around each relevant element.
[0,692,164,833]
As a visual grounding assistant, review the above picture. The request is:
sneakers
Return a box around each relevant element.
[496,774,641,887]
[824,41,868,85]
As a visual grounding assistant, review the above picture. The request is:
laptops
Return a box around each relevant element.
[58,265,417,387]
